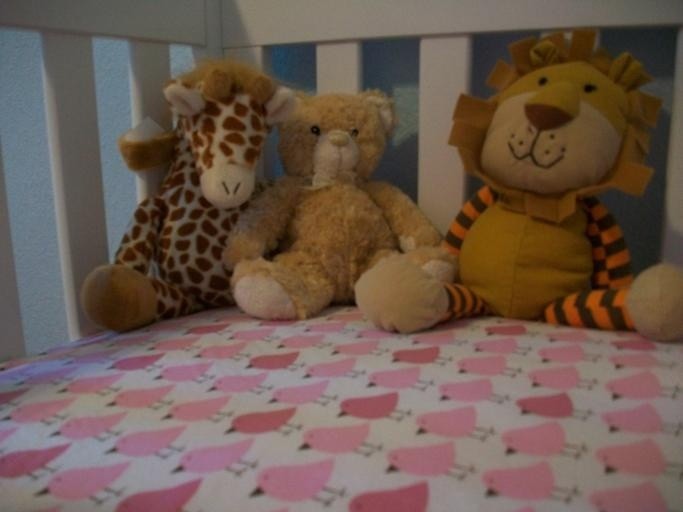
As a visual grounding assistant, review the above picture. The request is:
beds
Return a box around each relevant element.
[1,1,682,512]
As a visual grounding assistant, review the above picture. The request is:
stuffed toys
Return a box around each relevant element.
[221,86,461,322]
[354,24,683,340]
[84,56,308,334]
